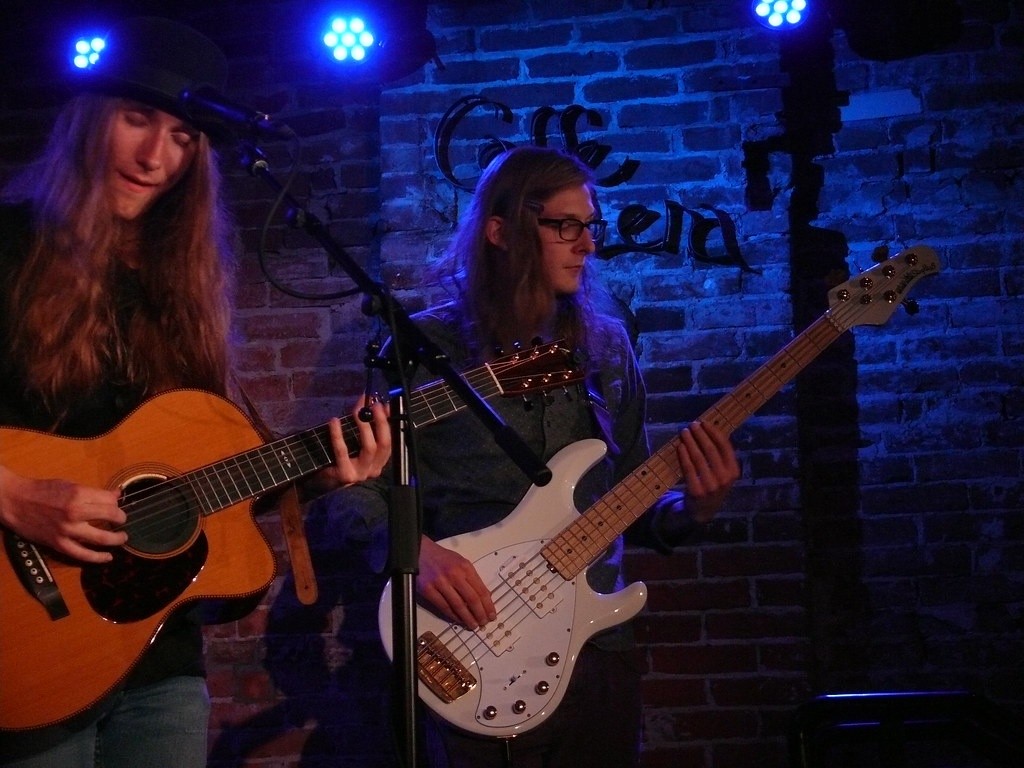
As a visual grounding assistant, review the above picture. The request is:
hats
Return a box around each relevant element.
[43,11,239,145]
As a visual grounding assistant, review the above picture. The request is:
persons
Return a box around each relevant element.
[316,148,742,768]
[0,16,392,768]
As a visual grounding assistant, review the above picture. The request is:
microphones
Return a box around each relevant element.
[183,82,294,141]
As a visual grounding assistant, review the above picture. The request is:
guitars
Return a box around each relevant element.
[374,236,945,741]
[0,324,590,741]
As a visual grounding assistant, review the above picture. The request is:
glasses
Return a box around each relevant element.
[538,217,609,242]
